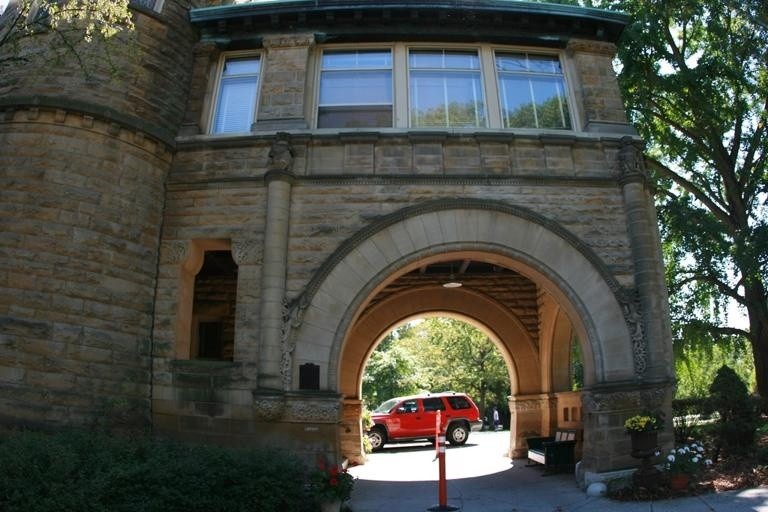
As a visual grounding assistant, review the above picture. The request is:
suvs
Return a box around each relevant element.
[365,390,481,452]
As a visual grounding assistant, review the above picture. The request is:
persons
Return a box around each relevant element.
[491,406,501,432]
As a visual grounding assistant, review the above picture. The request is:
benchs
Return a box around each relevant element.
[525,428,577,477]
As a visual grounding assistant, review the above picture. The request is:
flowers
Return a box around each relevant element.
[666,440,712,470]
[312,459,353,502]
[623,415,657,433]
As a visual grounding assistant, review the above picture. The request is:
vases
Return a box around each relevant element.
[321,499,342,511]
[669,470,690,487]
[630,431,657,451]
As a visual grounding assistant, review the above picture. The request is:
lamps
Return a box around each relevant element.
[441,263,463,288]
